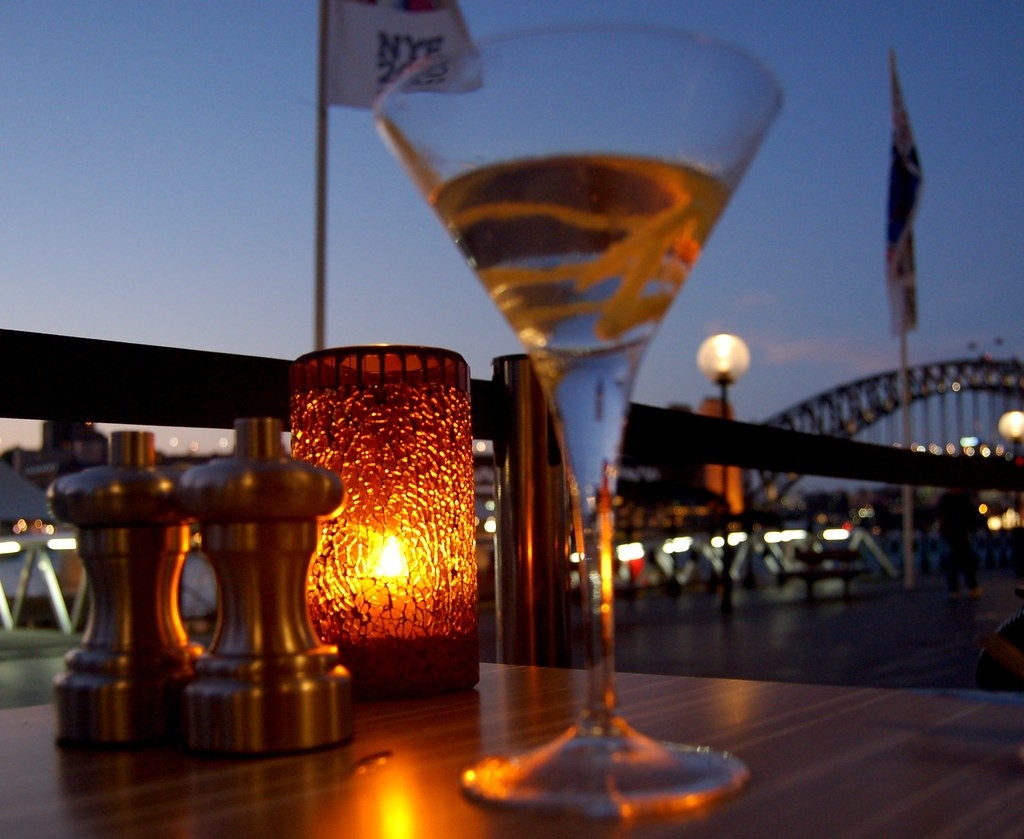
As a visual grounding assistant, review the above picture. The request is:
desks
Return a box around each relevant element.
[0,662,1024,839]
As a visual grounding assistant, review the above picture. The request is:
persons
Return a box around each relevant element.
[932,474,992,602]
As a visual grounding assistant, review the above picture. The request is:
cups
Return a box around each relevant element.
[286,346,479,697]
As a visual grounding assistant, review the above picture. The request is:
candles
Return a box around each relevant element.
[289,344,480,700]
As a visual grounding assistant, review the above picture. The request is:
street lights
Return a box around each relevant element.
[1000,411,1024,555]
[697,332,751,629]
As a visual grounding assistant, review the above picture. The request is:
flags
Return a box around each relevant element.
[320,0,483,110]
[885,51,924,338]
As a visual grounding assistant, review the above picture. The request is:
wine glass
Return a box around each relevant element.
[373,23,785,814]
